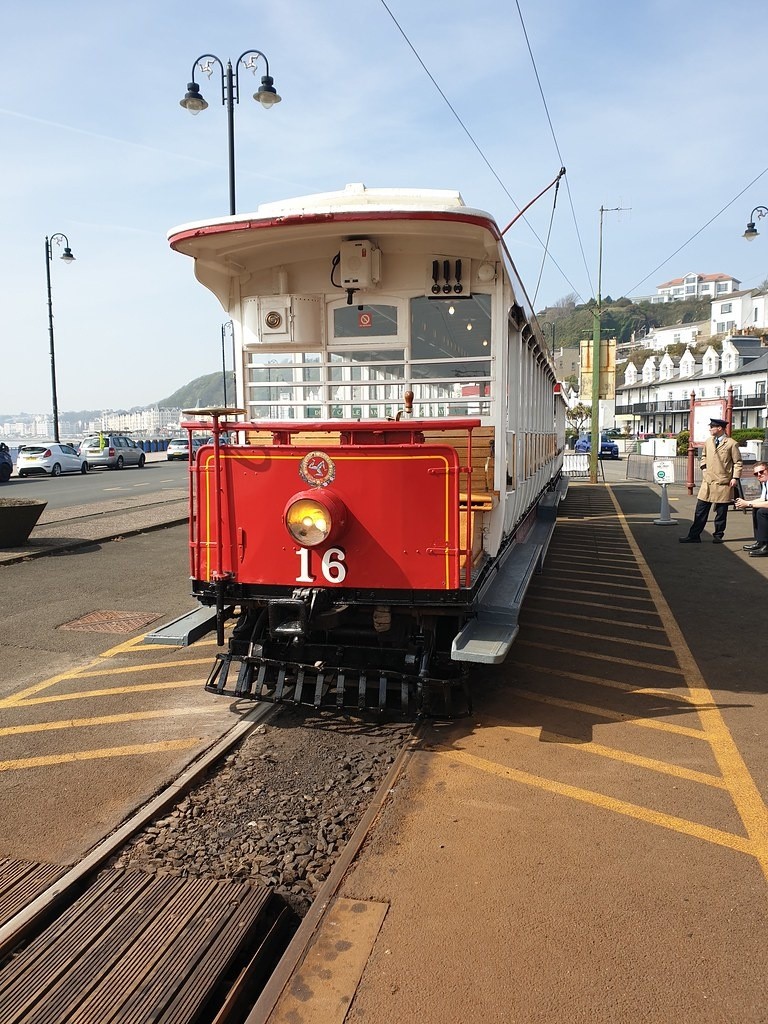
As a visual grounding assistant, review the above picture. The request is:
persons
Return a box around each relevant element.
[735,462,768,557]
[680,418,743,543]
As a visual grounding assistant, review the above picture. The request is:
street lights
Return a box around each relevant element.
[179,50,282,215]
[590,205,637,485]
[541,322,555,355]
[740,206,768,241]
[45,233,77,443]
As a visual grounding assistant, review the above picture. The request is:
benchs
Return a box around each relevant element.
[248,424,501,511]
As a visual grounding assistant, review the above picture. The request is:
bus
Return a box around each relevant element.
[168,183,571,720]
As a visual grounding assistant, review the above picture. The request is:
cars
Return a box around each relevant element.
[574,434,619,459]
[77,436,146,469]
[207,437,227,446]
[639,433,656,440]
[16,443,89,478]
[167,438,201,461]
[665,433,678,439]
[579,426,628,434]
[0,442,13,482]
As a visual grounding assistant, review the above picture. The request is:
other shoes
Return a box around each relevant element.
[679,536,701,543]
[713,536,721,543]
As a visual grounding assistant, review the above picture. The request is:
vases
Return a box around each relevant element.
[0,498,49,548]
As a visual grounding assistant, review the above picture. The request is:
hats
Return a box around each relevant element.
[708,418,729,428]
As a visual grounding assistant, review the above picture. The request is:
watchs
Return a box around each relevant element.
[749,504,752,507]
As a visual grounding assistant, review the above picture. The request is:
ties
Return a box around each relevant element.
[760,482,766,501]
[716,440,719,448]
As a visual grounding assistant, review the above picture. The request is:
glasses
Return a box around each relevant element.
[754,469,766,476]
[710,426,719,429]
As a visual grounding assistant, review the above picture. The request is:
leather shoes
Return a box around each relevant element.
[743,541,767,551]
[749,545,768,557]
